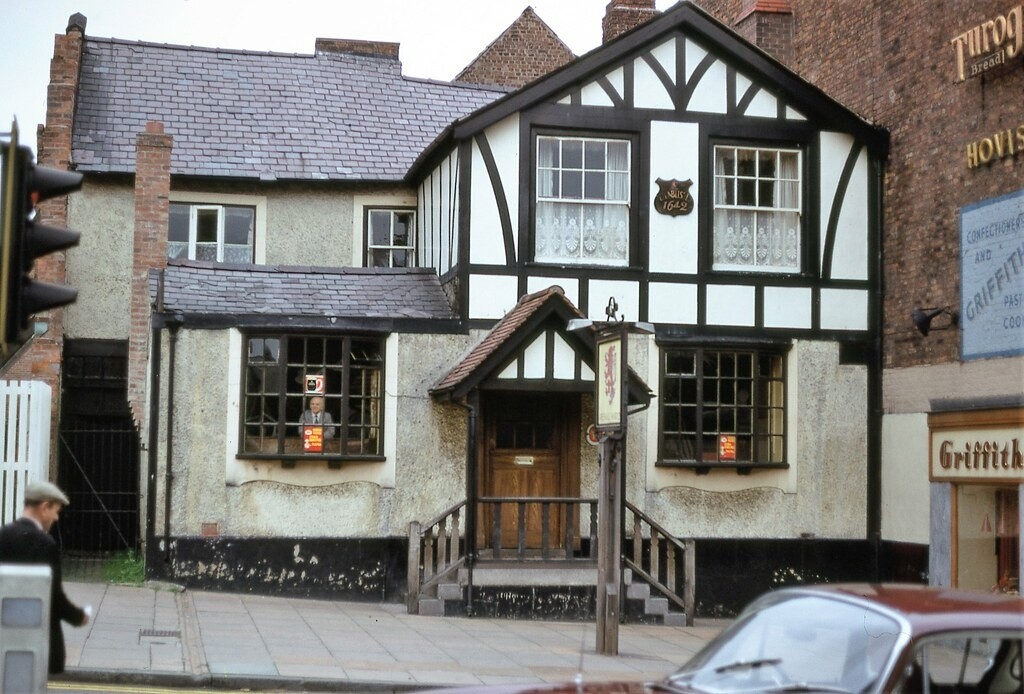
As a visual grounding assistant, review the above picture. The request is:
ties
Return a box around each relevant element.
[314,415,318,424]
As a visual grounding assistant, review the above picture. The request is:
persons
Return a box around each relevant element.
[0,481,90,678]
[297,396,336,440]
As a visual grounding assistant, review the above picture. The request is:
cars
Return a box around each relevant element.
[403,583,1024,694]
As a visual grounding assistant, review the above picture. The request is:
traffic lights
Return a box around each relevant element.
[0,116,83,385]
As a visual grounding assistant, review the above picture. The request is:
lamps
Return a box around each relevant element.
[910,304,960,336]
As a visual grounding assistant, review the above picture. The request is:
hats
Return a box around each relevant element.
[24,481,70,507]
